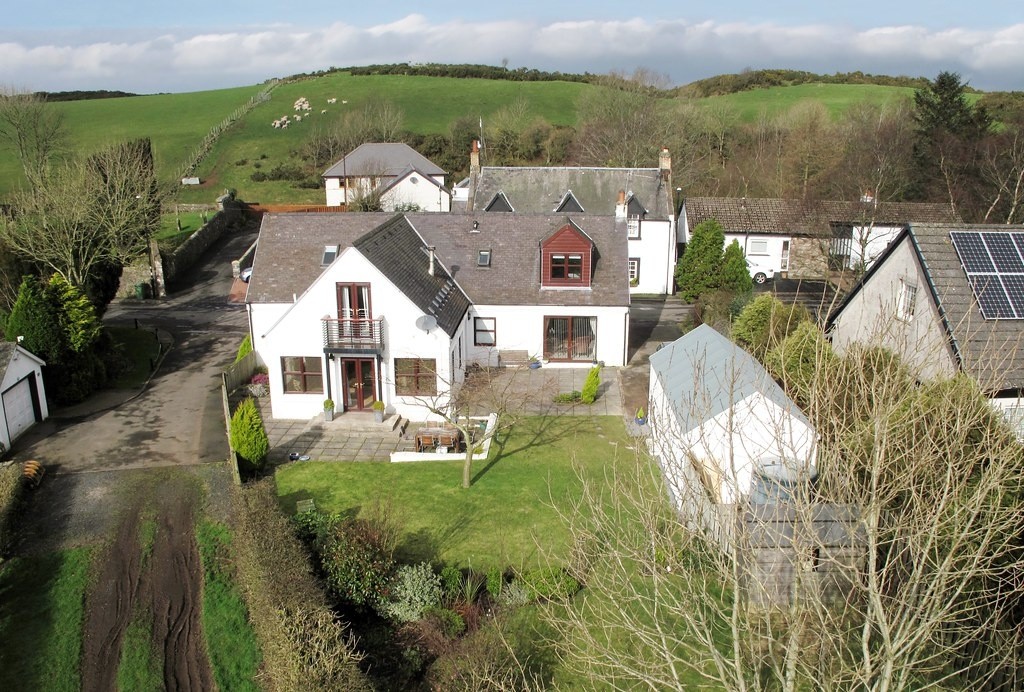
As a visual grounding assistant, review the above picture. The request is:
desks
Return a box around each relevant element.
[415,428,460,453]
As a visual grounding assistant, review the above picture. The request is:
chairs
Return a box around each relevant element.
[421,421,475,453]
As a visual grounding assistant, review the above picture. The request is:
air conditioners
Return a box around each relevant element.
[372,400,385,423]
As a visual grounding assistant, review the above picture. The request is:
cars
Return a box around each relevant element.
[745,257,775,285]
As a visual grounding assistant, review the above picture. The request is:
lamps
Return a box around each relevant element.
[329,353,335,361]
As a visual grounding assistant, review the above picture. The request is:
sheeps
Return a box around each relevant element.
[271,97,348,130]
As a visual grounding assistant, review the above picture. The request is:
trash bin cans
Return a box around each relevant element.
[135,282,145,300]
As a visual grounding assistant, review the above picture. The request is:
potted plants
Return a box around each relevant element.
[634,407,646,425]
[529,356,541,368]
[324,400,335,421]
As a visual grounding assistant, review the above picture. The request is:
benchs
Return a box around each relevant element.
[497,349,528,370]
